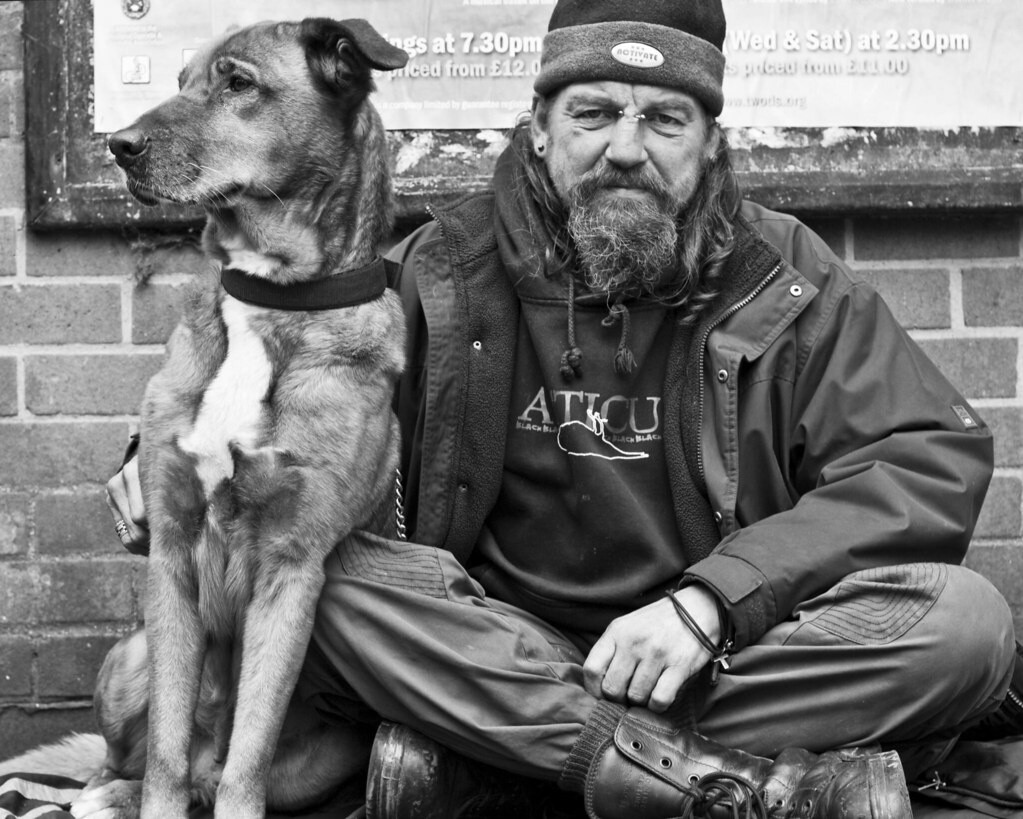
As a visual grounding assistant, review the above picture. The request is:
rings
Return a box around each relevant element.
[114,520,128,539]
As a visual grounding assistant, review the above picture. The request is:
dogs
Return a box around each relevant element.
[1,15,412,819]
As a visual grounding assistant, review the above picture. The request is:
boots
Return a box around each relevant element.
[364,719,538,819]
[558,699,914,819]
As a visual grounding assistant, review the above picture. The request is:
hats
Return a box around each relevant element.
[533,0,727,117]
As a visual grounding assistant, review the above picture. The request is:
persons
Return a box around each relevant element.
[105,0,1018,819]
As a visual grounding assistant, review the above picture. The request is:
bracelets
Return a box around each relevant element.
[664,586,734,673]
